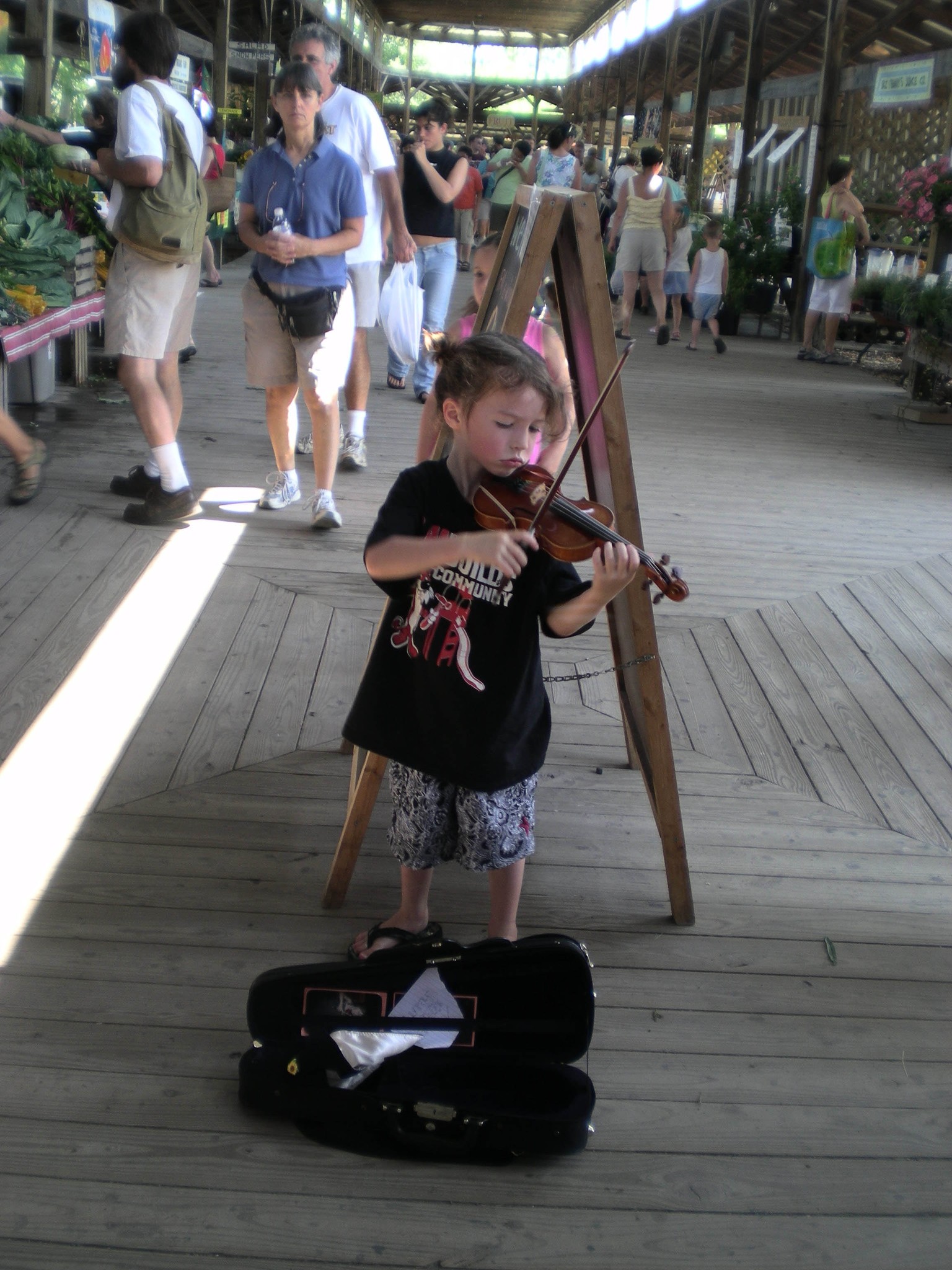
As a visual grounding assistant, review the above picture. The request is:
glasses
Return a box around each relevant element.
[474,141,483,145]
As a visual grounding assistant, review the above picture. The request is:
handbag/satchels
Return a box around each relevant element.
[378,254,426,364]
[276,284,342,340]
[805,191,858,279]
[603,166,620,199]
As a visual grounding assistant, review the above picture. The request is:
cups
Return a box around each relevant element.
[881,250,893,276]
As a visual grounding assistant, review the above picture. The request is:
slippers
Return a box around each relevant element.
[656,324,670,345]
[714,337,727,354]
[686,342,697,350]
[197,279,219,288]
[419,391,430,404]
[217,279,222,284]
[349,920,442,962]
[387,372,406,389]
[615,328,631,340]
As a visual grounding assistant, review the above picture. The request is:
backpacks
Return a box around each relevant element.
[112,82,208,264]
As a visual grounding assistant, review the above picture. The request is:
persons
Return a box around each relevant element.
[65,8,206,526]
[381,92,728,404]
[236,60,367,529]
[415,234,580,474]
[289,22,418,468]
[797,158,870,366]
[348,330,640,960]
[0,408,52,503]
[0,91,227,365]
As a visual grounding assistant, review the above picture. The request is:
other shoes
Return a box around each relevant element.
[821,352,853,364]
[797,345,827,361]
[178,340,197,363]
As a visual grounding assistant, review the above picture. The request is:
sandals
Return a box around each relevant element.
[646,326,659,335]
[457,260,462,269]
[461,261,471,271]
[9,437,52,505]
[670,331,681,340]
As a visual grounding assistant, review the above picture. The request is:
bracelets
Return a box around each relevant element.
[12,117,19,129]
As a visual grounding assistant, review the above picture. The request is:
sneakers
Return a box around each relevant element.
[123,486,203,524]
[259,471,301,509]
[111,465,160,498]
[340,432,367,469]
[296,424,345,453]
[302,492,342,529]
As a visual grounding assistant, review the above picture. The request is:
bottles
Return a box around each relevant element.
[272,207,295,265]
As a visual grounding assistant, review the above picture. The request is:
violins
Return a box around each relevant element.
[470,463,690,607]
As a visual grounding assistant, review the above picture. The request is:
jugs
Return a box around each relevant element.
[867,247,883,280]
